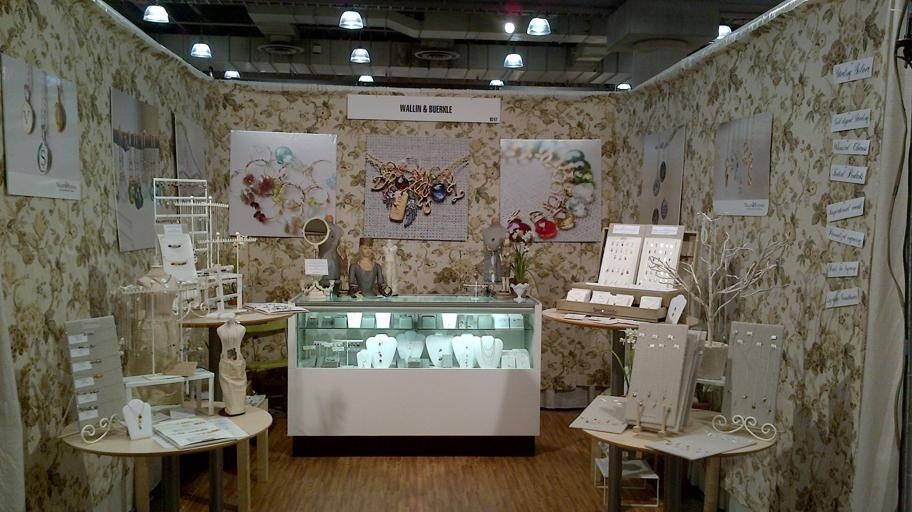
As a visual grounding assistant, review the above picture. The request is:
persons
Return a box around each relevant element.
[316,214,345,285]
[346,235,393,300]
[472,216,508,281]
[215,312,248,416]
[381,238,399,297]
[499,230,514,293]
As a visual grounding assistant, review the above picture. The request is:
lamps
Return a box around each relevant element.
[359,73,375,82]
[707,17,731,44]
[618,80,631,90]
[224,64,240,78]
[191,41,212,58]
[339,10,363,29]
[504,52,523,68]
[527,15,552,35]
[142,1,169,24]
[350,48,370,64]
[489,79,505,86]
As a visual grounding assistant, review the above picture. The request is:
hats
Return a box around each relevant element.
[359,237,373,247]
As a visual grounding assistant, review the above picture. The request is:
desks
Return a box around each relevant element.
[128,302,295,512]
[60,401,273,512]
[134,395,269,512]
[543,308,699,409]
[582,409,776,511]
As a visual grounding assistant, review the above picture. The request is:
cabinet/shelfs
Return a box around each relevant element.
[287,292,543,457]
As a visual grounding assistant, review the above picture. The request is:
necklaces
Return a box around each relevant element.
[404,333,420,356]
[481,339,496,366]
[363,148,469,230]
[126,400,147,433]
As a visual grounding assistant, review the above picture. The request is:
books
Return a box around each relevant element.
[152,417,235,449]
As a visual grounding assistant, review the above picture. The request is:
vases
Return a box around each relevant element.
[697,342,728,380]
[692,400,712,409]
[510,283,529,303]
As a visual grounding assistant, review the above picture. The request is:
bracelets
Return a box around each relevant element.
[228,143,335,235]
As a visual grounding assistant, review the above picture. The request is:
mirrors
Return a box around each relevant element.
[303,217,331,258]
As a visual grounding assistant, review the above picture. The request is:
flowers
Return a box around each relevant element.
[611,328,638,396]
[506,218,533,285]
[695,383,713,402]
